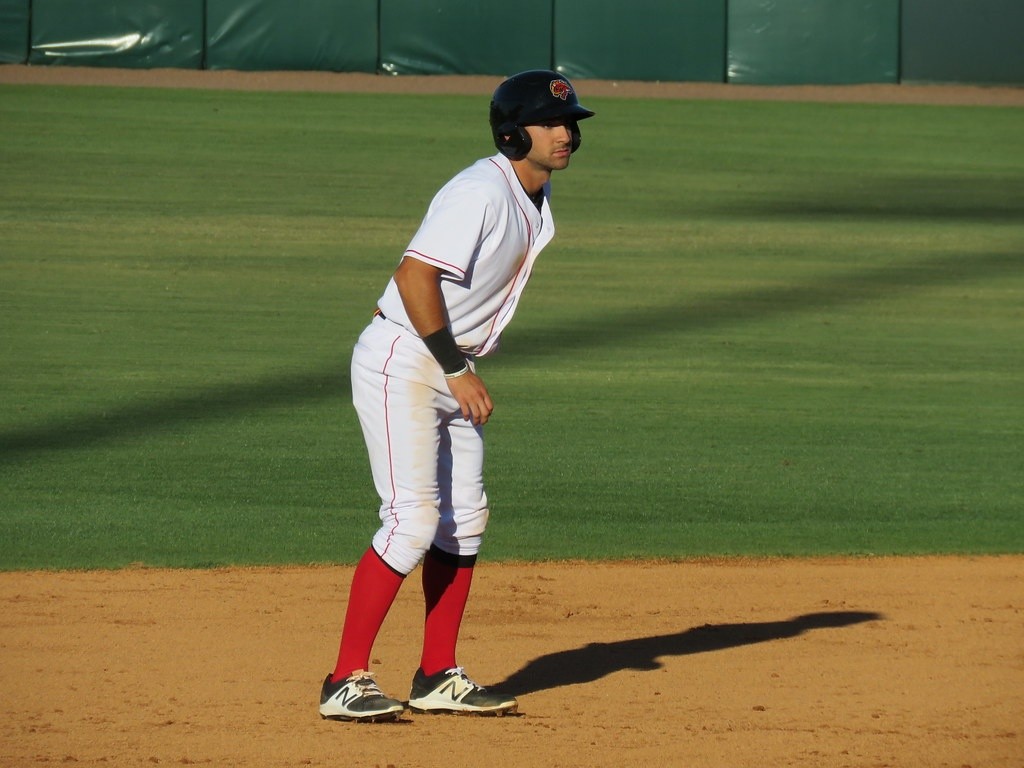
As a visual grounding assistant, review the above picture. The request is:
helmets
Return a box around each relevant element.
[489,69,597,161]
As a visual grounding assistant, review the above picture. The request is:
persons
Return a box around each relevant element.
[319,70,597,721]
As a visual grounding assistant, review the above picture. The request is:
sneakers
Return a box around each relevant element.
[320,669,404,722]
[408,665,518,717]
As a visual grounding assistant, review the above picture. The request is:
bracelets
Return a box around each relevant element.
[444,364,469,379]
